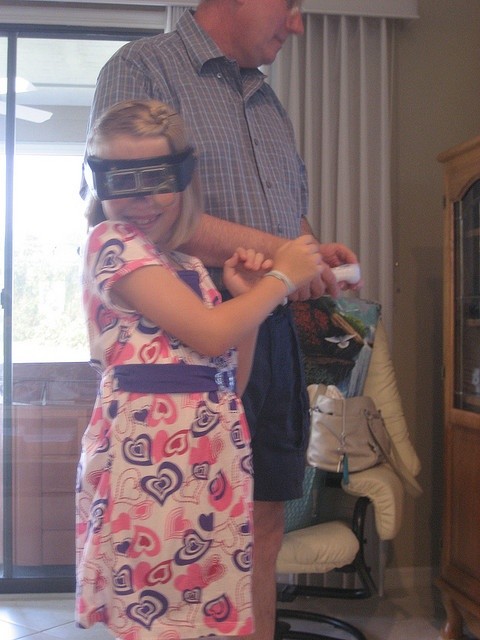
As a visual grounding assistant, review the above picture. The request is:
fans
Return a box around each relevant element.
[0,76,53,124]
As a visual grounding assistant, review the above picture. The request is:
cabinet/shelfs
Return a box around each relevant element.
[439,136,480,638]
[12,402,92,567]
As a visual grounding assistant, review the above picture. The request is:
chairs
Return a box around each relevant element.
[270,324,420,640]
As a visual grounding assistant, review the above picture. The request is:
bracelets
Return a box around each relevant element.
[266,269,293,296]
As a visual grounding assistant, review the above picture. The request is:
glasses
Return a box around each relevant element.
[84,148,194,201]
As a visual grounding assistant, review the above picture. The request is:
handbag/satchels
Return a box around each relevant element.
[307,395,391,475]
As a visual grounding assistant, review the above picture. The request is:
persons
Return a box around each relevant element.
[76,103,324,640]
[80,0,362,640]
